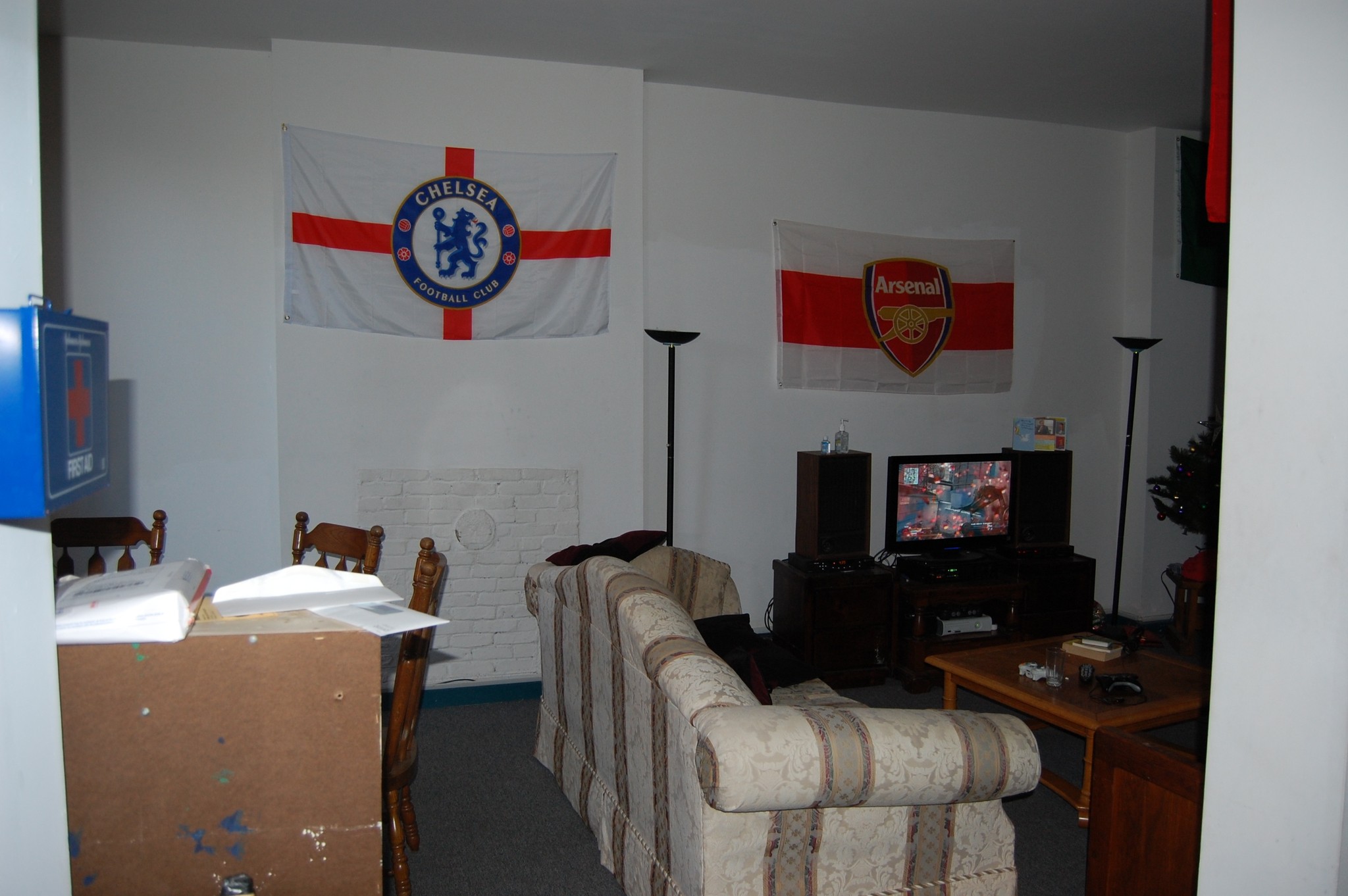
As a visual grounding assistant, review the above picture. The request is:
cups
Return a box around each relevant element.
[1045,646,1066,687]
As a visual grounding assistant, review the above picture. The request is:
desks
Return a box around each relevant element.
[772,555,895,688]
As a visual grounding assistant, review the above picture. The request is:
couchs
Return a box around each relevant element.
[525,545,1044,896]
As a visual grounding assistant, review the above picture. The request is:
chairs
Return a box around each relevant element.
[382,536,446,895]
[51,507,166,580]
[290,512,382,576]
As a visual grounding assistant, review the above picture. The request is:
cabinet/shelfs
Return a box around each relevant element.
[897,552,1095,695]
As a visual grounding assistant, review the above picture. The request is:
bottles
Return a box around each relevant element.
[821,436,831,454]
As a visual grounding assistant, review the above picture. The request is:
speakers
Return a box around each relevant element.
[795,449,872,560]
[1001,446,1073,547]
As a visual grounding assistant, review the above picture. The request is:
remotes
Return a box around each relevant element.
[1079,664,1093,683]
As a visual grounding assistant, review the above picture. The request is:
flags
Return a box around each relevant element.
[281,122,617,340]
[772,218,1014,395]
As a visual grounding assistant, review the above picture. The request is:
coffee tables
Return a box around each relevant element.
[925,631,1207,828]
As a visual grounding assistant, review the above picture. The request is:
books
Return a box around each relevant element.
[1062,637,1123,662]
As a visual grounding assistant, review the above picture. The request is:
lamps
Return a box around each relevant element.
[1111,336,1165,616]
[645,328,702,551]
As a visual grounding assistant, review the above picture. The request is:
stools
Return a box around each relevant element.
[1164,562,1206,650]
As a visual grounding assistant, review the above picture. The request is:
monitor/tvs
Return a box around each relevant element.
[884,451,1018,560]
